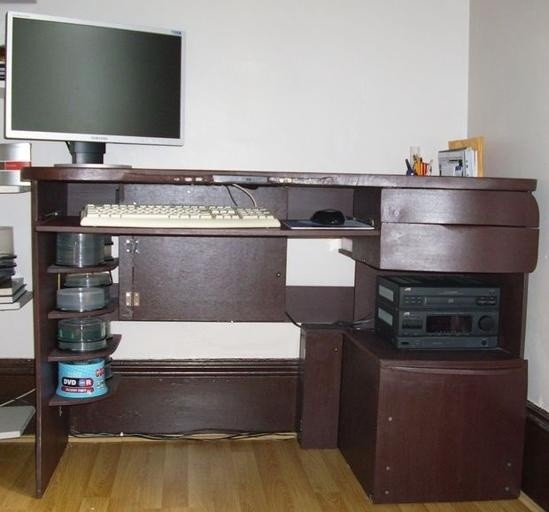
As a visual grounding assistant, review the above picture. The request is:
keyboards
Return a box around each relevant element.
[80,202,280,229]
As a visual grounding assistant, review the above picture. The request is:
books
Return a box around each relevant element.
[0,253,34,312]
[0,405,36,439]
[437,136,485,178]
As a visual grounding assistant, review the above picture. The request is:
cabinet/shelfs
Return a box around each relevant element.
[20,166,539,505]
[0,79,36,438]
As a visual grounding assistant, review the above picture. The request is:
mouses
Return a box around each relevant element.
[312,208,344,226]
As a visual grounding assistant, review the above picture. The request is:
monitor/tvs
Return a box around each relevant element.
[4,10,188,169]
